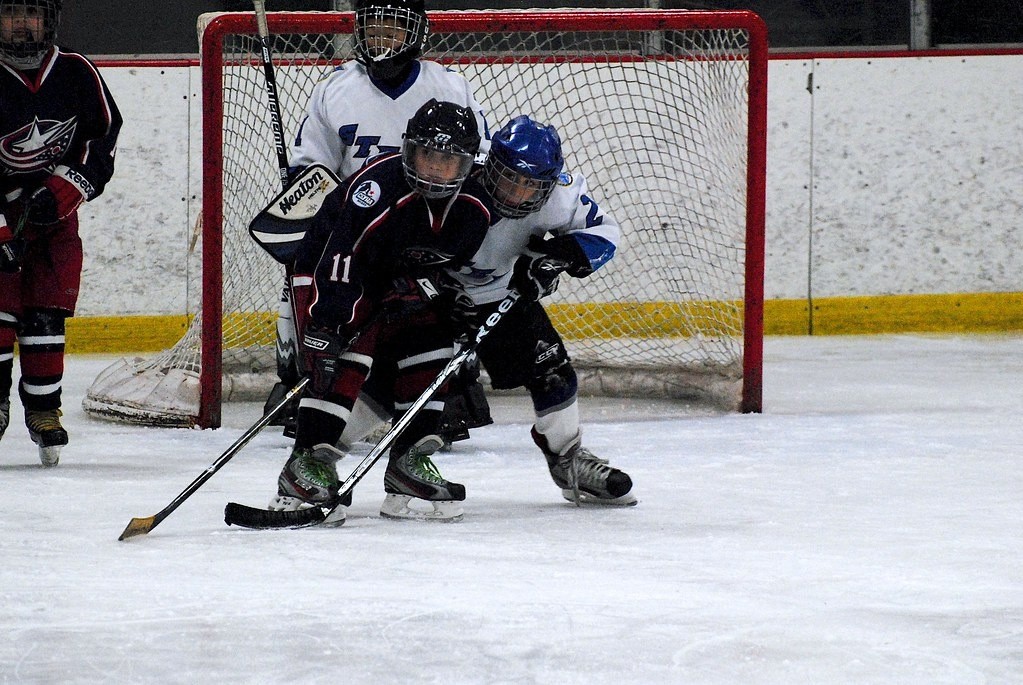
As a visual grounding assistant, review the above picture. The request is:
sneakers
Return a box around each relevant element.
[529,425,636,506]
[379,434,467,522]
[17,379,70,468]
[0,382,9,441]
[267,436,349,527]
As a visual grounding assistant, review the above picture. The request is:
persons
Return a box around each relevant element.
[0,0,124,466]
[334,115,640,501]
[265,1,493,451]
[269,98,492,522]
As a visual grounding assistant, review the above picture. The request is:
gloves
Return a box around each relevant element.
[0,214,23,278]
[512,233,586,303]
[370,264,440,325]
[24,165,93,225]
[284,316,362,395]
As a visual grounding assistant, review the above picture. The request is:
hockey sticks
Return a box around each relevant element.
[118,303,391,541]
[225,288,522,531]
[252,0,290,191]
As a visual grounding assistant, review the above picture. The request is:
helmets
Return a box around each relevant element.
[354,0,427,77]
[0,0,56,68]
[483,116,565,219]
[401,98,480,199]
